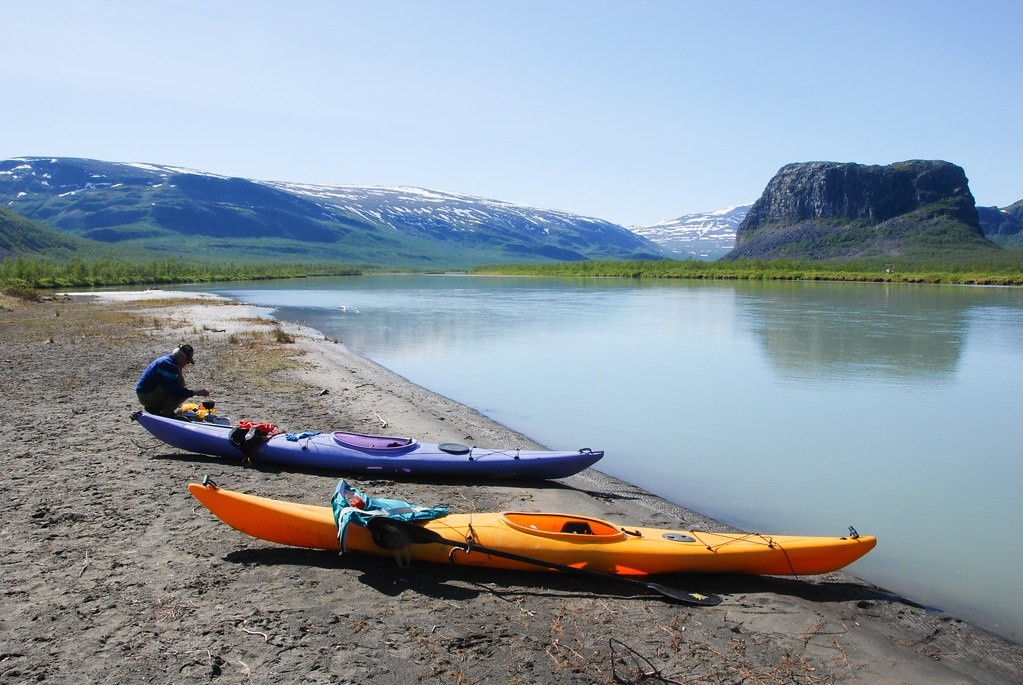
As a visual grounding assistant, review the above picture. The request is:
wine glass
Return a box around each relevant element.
[202,395,216,422]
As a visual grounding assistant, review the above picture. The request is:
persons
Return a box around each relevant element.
[137,344,208,419]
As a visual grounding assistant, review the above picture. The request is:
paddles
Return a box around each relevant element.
[366,516,722,608]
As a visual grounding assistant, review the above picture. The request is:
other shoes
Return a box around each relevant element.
[161,410,177,418]
[145,407,160,415]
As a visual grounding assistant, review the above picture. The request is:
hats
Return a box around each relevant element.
[178,344,195,364]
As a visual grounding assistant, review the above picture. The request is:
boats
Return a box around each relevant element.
[186,475,878,579]
[129,404,605,483]
